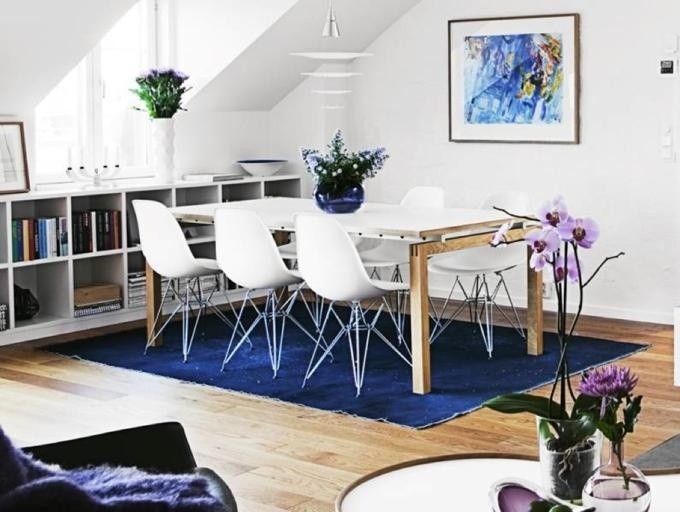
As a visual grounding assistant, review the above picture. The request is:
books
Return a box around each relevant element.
[10,205,120,266]
[183,172,245,181]
[128,263,219,308]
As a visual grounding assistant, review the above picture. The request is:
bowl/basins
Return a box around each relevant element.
[234,158,290,176]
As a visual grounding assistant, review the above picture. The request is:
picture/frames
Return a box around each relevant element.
[444,11,583,150]
[0,119,33,196]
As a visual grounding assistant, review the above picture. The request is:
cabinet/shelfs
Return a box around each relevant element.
[0,173,303,348]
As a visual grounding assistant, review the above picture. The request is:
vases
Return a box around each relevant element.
[150,121,176,179]
[312,182,366,217]
[531,404,600,499]
[581,441,651,511]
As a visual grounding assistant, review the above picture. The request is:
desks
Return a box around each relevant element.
[332,452,679,511]
[170,195,547,396]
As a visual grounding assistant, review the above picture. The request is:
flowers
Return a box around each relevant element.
[491,197,627,448]
[297,125,388,194]
[128,64,192,122]
[574,362,645,491]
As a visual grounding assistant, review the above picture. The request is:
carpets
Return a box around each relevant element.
[32,297,651,429]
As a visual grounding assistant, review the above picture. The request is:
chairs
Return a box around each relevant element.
[0,419,240,510]
